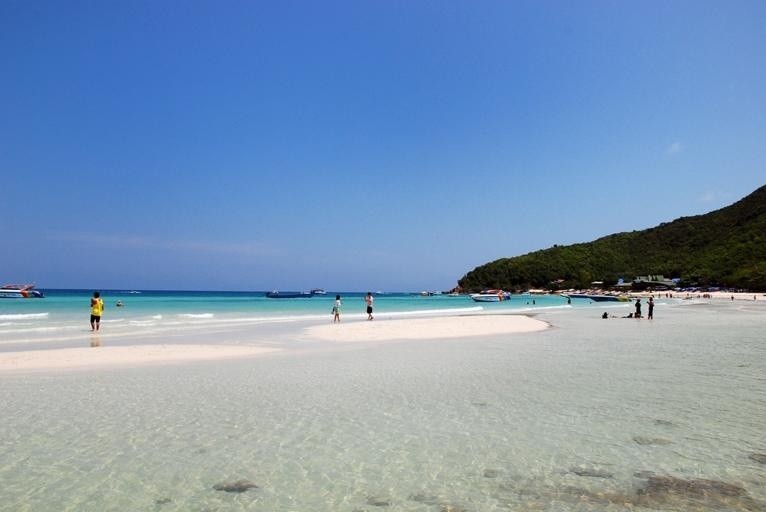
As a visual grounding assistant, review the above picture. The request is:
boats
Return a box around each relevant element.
[420,289,511,302]
[560,292,632,302]
[266,288,327,298]
[0,281,44,298]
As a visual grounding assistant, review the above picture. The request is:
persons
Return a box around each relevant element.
[333,295,342,323]
[646,297,654,319]
[89,291,104,332]
[116,300,122,307]
[635,298,641,318]
[365,292,374,320]
